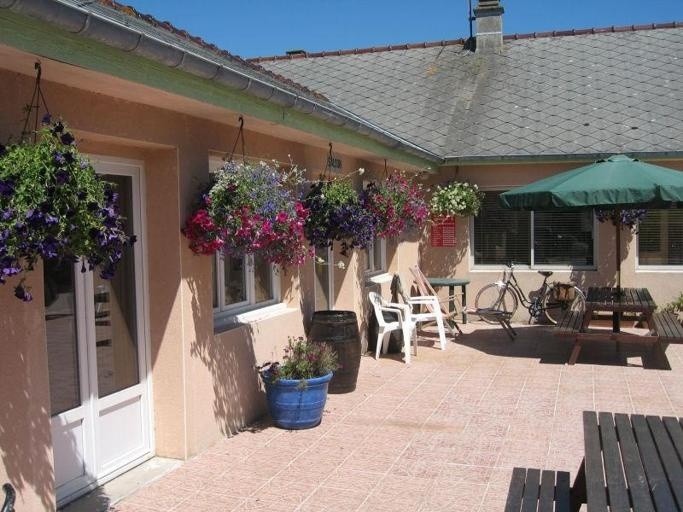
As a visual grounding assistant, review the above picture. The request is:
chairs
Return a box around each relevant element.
[408,264,517,342]
[366,289,418,361]
[394,290,448,351]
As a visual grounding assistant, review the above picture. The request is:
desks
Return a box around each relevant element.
[568,284,658,369]
[425,277,470,327]
[574,407,681,512]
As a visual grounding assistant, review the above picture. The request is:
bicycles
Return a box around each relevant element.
[472,256,586,327]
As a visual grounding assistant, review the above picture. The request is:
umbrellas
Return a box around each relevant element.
[497,154,683,350]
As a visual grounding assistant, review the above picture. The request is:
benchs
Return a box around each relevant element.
[555,309,586,365]
[647,310,682,370]
[499,463,572,511]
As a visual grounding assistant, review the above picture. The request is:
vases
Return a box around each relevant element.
[260,365,334,429]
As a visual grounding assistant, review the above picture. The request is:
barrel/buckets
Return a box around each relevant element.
[306,310,361,393]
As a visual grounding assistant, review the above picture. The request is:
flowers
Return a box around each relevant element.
[254,332,341,388]
